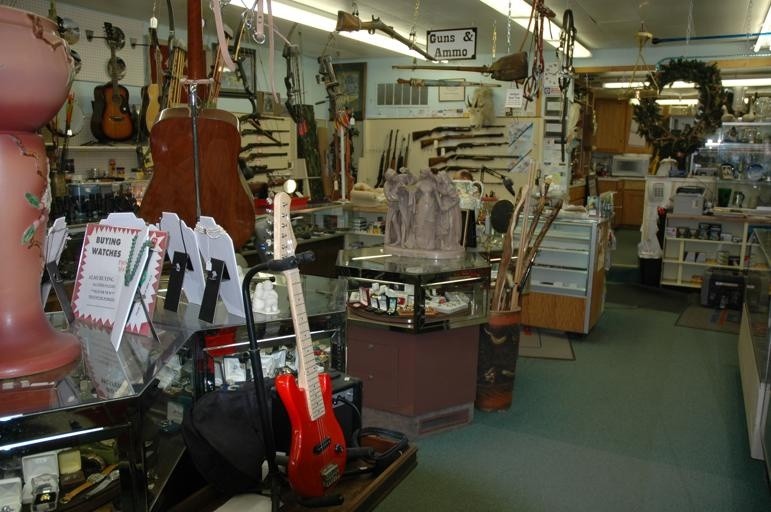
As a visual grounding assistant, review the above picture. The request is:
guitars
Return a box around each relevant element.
[262,175,346,502]
[95,23,225,173]
[140,0,256,255]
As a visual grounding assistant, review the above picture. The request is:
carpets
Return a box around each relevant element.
[674,302,770,339]
[520,327,578,362]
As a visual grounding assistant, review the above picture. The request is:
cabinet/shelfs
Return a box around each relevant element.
[241,202,386,258]
[594,100,629,154]
[599,181,624,227]
[661,212,770,292]
[624,180,645,227]
[473,214,614,339]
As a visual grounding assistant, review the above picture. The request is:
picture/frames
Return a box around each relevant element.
[329,61,367,122]
[211,42,258,99]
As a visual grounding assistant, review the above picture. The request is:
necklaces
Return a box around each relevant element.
[195,222,227,240]
[124,234,153,286]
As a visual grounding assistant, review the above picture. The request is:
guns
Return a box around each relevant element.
[335,10,436,62]
[412,125,521,175]
[371,128,410,188]
[238,112,291,179]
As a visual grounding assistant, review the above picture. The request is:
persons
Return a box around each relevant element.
[719,126,749,167]
[384,166,463,249]
[453,169,488,198]
